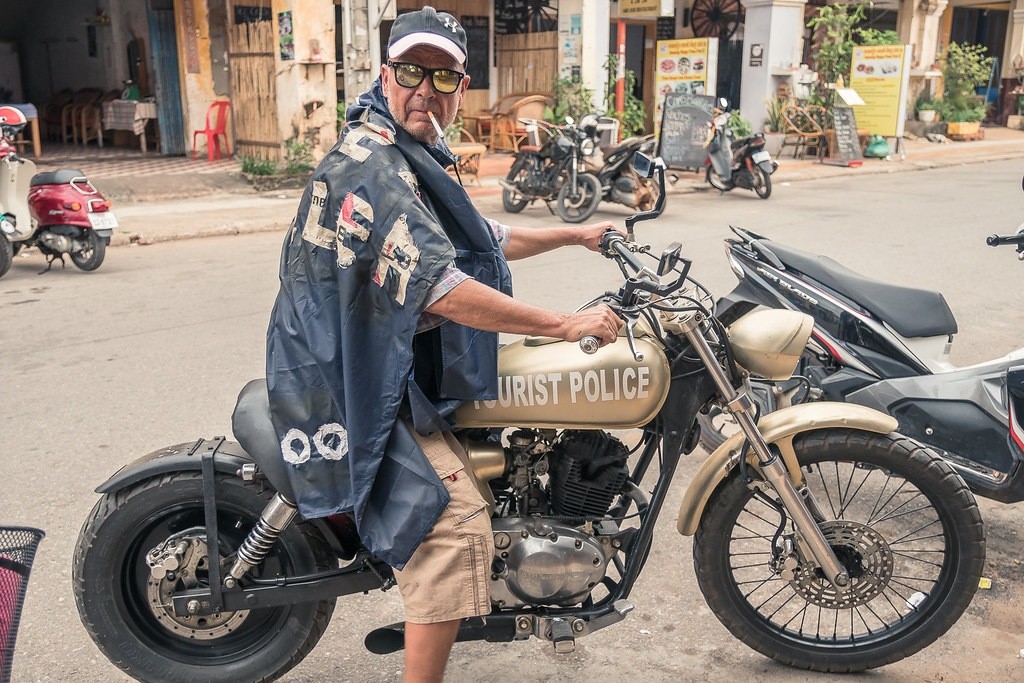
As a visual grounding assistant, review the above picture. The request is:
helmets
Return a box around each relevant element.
[0,106,27,136]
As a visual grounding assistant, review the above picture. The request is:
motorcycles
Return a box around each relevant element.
[497,114,665,223]
[67,151,986,683]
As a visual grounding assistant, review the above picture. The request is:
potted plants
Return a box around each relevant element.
[943,97,986,134]
[918,102,936,122]
[762,92,802,155]
[240,138,312,191]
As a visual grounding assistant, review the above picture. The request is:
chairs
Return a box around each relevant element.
[42,89,75,144]
[477,93,554,153]
[444,126,487,190]
[60,88,102,144]
[799,104,832,162]
[81,89,122,146]
[775,104,825,159]
[193,99,231,162]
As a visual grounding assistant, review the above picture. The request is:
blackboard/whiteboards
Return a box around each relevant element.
[459,15,490,90]
[831,106,864,161]
[658,92,715,167]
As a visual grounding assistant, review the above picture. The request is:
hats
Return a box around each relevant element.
[389,5,469,66]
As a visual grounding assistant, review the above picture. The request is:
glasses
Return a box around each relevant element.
[388,59,467,95]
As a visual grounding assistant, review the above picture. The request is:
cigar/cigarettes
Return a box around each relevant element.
[427,111,444,140]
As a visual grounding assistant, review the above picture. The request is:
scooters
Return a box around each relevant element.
[0,106,120,277]
[703,97,777,199]
[714,175,1024,504]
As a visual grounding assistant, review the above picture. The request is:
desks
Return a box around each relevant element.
[95,100,160,152]
[462,114,501,152]
[0,103,41,157]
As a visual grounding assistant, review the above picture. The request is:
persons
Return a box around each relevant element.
[266,7,629,682]
